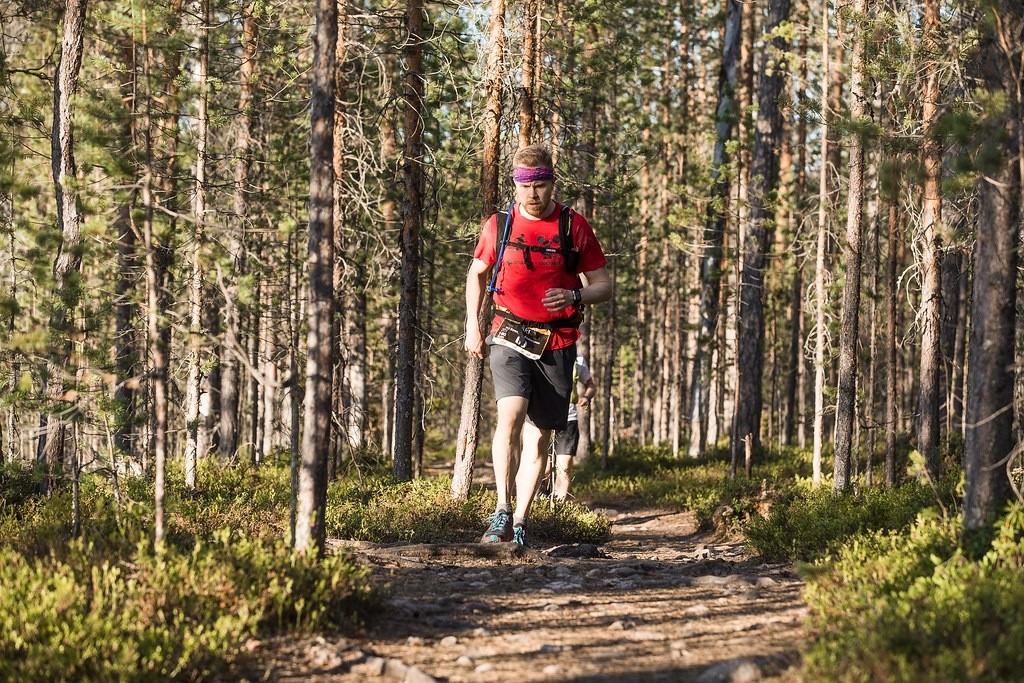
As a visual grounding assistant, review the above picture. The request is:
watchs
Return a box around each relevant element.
[571,288,581,307]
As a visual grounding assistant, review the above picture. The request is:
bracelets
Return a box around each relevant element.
[582,396,590,401]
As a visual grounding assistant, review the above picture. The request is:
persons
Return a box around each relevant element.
[464,145,612,545]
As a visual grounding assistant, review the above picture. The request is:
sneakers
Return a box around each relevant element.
[510,523,527,545]
[482,509,513,542]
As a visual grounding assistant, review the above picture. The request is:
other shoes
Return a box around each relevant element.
[534,475,548,503]
